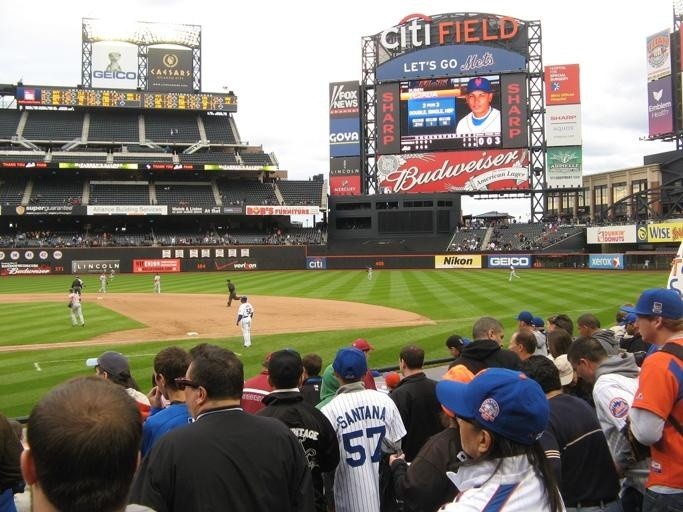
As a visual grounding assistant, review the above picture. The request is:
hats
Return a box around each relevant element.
[446,334,472,347]
[433,363,551,446]
[84,351,131,380]
[462,77,494,94]
[239,296,247,301]
[350,338,375,351]
[268,348,303,384]
[512,311,545,328]
[619,313,636,326]
[332,347,368,379]
[383,370,401,387]
[619,287,683,318]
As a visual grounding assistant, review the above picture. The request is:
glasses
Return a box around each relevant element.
[174,376,199,392]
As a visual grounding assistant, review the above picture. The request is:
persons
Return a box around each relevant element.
[98,272,106,293]
[367,265,372,279]
[446,217,577,252]
[225,279,241,307]
[151,272,160,293]
[507,264,520,281]
[71,276,80,301]
[453,76,501,136]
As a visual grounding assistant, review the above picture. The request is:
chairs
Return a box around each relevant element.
[448,221,582,250]
[1,105,327,250]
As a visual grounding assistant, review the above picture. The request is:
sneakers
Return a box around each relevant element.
[72,322,85,327]
